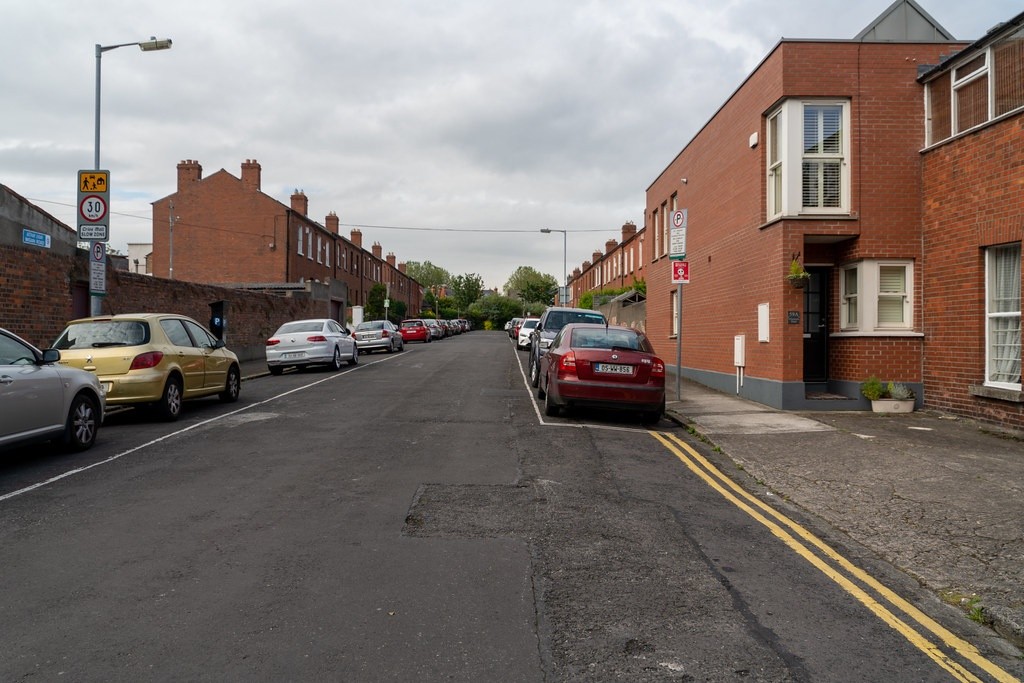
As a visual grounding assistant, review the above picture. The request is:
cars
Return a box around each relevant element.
[0,327,109,457]
[265,318,357,376]
[44,313,240,427]
[398,318,475,344]
[503,317,541,351]
[537,322,666,427]
[351,320,403,354]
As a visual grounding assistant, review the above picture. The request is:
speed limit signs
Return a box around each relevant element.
[80,194,107,222]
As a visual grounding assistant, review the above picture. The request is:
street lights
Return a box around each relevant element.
[88,35,172,319]
[540,228,567,309]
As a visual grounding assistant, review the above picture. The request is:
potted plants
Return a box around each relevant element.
[784,258,811,289]
[859,373,916,414]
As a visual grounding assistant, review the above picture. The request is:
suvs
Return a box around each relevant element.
[529,307,609,388]
